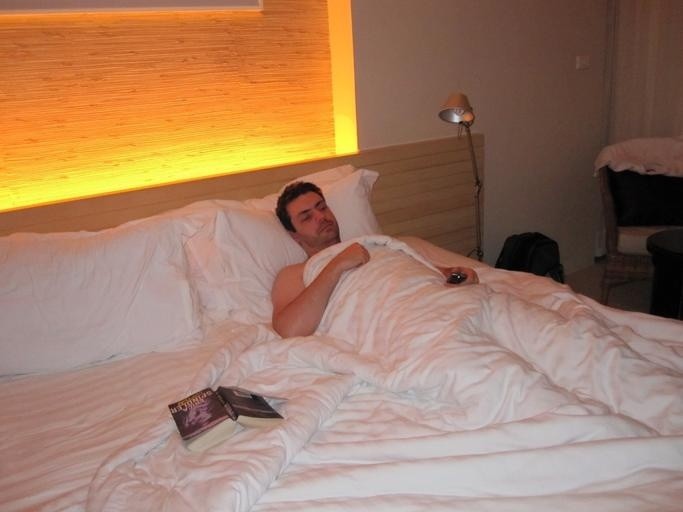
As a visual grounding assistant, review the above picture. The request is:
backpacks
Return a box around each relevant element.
[494,232,563,283]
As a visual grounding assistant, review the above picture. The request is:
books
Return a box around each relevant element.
[167,385,284,453]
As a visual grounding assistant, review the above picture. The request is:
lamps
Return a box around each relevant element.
[438,94,484,259]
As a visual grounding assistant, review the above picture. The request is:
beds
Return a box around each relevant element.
[1,136,682,512]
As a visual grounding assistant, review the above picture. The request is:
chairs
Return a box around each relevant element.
[595,139,681,307]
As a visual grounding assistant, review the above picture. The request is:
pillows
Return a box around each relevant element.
[172,165,382,331]
[5,209,217,376]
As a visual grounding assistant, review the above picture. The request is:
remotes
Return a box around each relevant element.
[447,273,467,284]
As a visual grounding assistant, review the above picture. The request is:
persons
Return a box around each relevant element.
[272,180,479,341]
